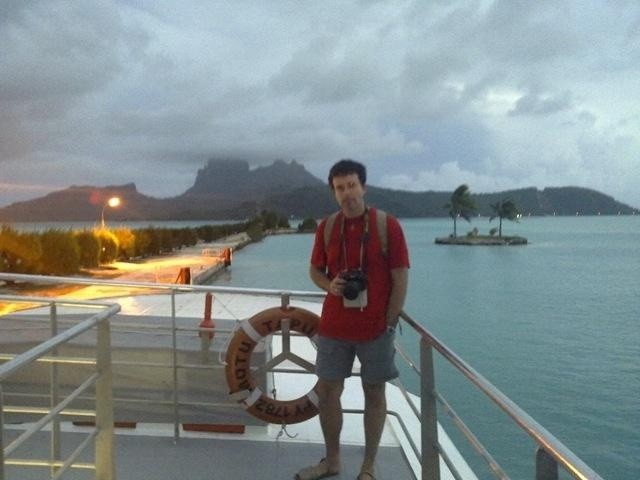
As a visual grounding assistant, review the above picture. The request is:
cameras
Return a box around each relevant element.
[338,270,367,300]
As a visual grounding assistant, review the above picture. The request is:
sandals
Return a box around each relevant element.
[296,458,338,479]
[358,469,376,480]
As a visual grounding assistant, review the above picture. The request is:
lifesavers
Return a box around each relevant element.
[222,306,321,425]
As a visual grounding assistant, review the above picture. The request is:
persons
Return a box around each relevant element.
[294,160,410,480]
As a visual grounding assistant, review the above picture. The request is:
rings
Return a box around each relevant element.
[335,288,337,292]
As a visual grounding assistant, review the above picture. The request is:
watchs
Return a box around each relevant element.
[386,326,396,336]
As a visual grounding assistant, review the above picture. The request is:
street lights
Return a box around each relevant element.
[102,198,120,226]
